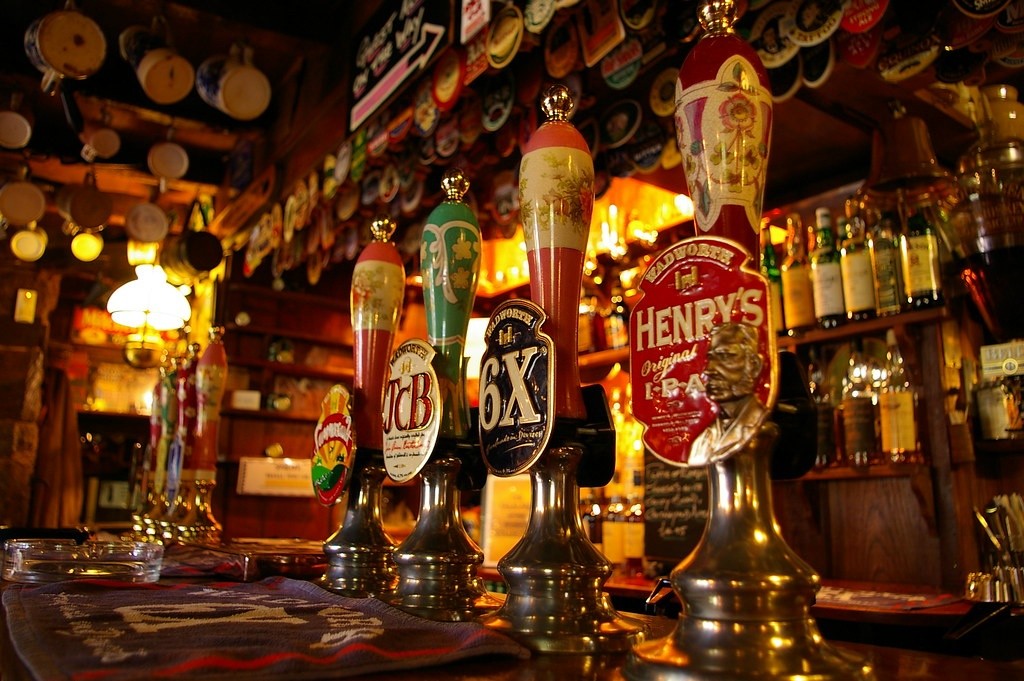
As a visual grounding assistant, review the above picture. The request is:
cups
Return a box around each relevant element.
[79,121,120,161]
[147,129,189,193]
[126,188,168,244]
[119,26,195,105]
[25,0,107,90]
[0,92,35,148]
[158,201,224,286]
[56,173,112,236]
[197,45,272,121]
[0,166,47,226]
[10,221,48,261]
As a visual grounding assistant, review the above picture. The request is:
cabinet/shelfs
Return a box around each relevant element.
[214,280,957,539]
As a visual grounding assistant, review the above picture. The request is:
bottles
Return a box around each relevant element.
[758,189,942,337]
[577,286,629,354]
[842,340,876,467]
[879,331,926,468]
[806,351,834,467]
